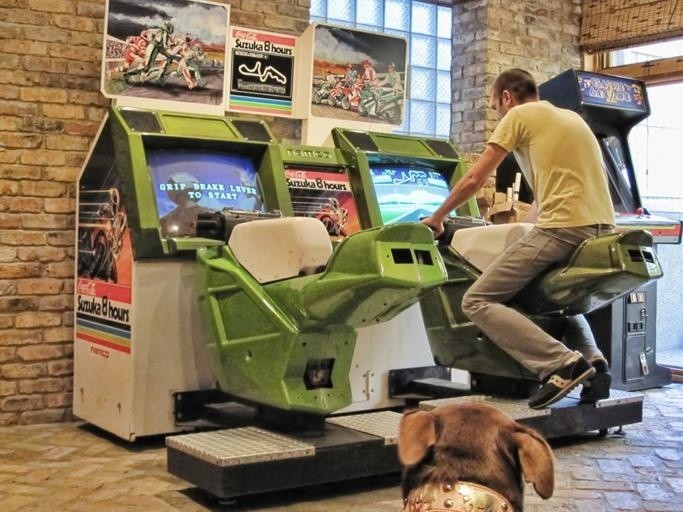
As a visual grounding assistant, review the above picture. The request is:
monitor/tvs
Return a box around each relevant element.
[369,163,459,227]
[590,125,639,214]
[144,144,267,238]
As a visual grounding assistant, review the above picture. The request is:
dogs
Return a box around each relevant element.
[395,403,555,512]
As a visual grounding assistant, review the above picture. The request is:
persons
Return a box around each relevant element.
[81,203,118,283]
[420,69,616,409]
[326,69,339,88]
[368,61,404,98]
[107,22,208,91]
[360,60,376,81]
[344,63,358,81]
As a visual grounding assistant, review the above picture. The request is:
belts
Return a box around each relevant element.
[589,223,614,229]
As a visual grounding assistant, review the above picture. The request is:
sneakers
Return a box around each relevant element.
[528,350,595,408]
[579,355,612,402]
